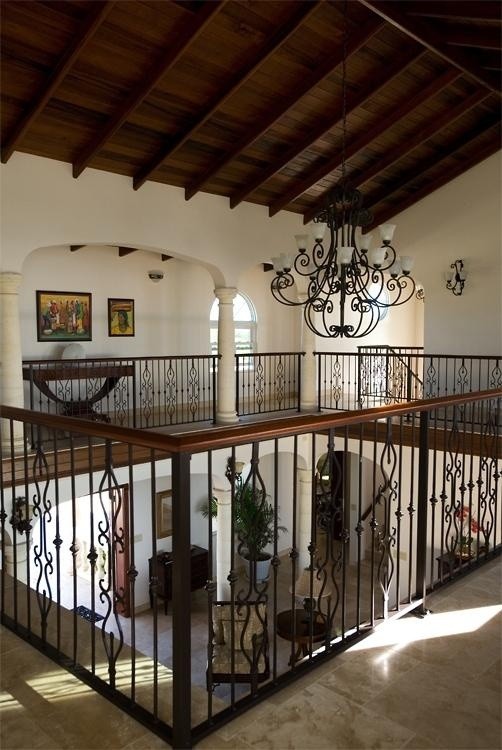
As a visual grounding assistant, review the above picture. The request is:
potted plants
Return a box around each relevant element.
[231,484,288,585]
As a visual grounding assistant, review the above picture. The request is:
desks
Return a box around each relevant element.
[23,364,136,426]
[274,608,336,666]
[436,551,463,581]
[147,545,210,616]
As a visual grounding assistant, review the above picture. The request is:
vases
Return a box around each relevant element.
[453,535,472,560]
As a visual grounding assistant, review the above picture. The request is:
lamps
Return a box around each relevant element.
[148,269,162,283]
[269,43,427,337]
[444,259,468,298]
[8,498,39,534]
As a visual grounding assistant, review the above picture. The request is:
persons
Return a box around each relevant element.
[43,299,84,336]
[111,310,133,335]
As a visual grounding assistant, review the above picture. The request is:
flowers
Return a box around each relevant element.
[449,505,482,539]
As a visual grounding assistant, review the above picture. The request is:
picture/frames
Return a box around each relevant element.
[154,487,172,540]
[35,289,136,342]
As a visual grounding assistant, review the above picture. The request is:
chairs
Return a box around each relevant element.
[206,599,270,695]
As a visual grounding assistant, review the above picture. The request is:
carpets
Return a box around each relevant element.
[70,605,107,626]
